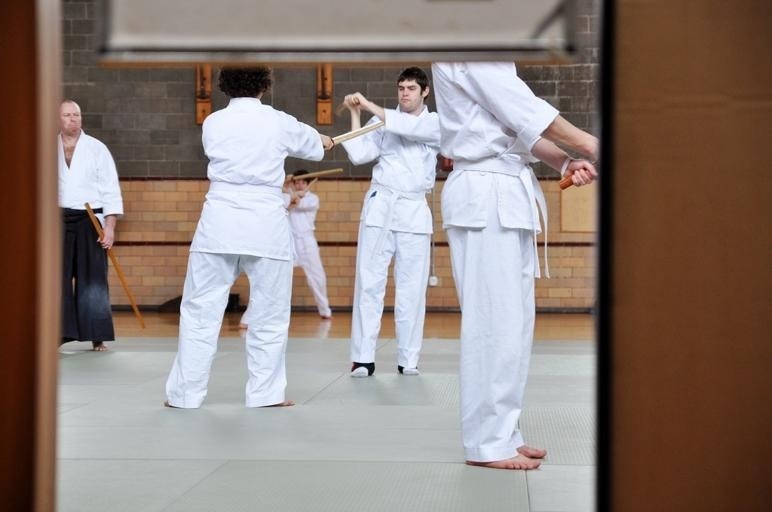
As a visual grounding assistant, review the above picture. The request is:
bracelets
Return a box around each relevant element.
[558,156,574,178]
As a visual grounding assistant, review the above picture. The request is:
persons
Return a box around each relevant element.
[57,96,126,353]
[281,167,334,320]
[338,64,443,381]
[429,62,603,472]
[162,64,337,410]
[237,188,306,330]
